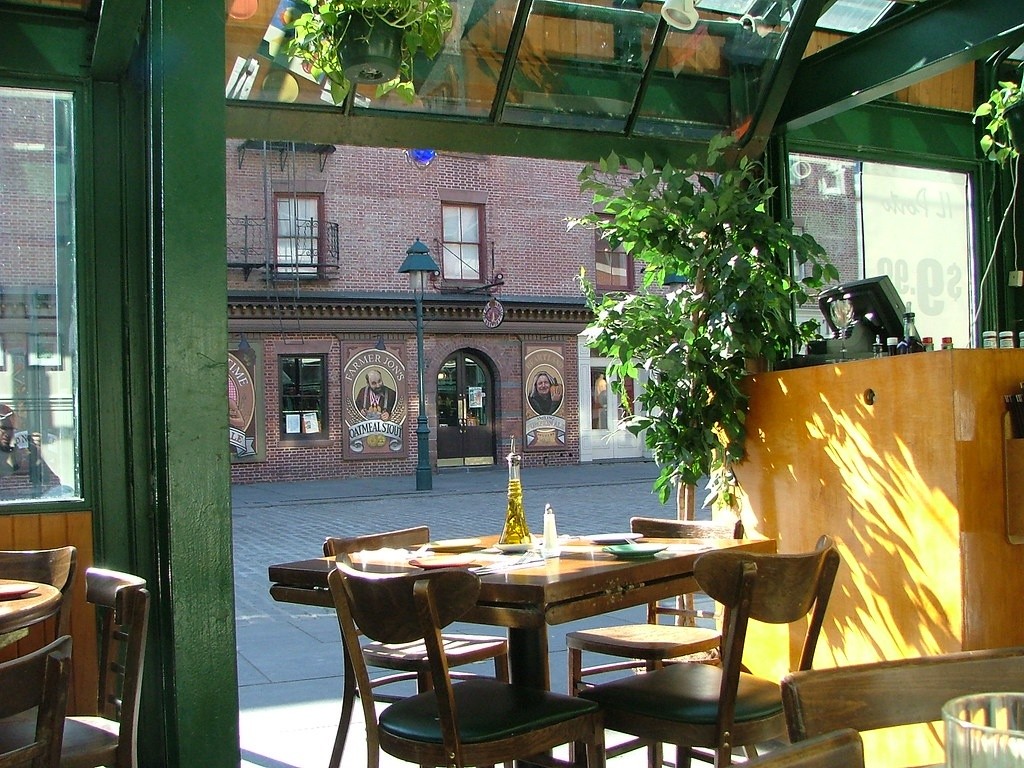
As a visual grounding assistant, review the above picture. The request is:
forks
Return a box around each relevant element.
[625,537,637,544]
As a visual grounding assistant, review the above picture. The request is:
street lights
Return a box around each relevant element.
[397,237,440,491]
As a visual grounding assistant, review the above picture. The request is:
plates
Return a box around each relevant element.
[425,539,481,551]
[602,543,669,559]
[0,584,39,600]
[494,544,536,552]
[409,555,475,568]
[592,533,644,543]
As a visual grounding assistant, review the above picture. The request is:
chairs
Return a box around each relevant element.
[327,549,606,767]
[323,527,507,767]
[0,545,150,768]
[575,533,840,767]
[783,643,1024,742]
[567,515,753,768]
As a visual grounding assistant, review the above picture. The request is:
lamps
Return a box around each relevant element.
[376,334,389,351]
[494,273,505,285]
[428,269,440,281]
[235,333,251,353]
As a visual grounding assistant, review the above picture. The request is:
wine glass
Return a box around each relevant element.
[830,299,854,352]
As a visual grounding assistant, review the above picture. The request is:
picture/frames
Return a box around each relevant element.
[24,334,64,371]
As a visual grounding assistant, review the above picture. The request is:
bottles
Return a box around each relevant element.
[499,434,531,545]
[886,337,898,356]
[1019,331,1024,348]
[872,335,884,358]
[543,501,561,559]
[923,336,933,351]
[999,330,1014,349]
[897,301,924,357]
[982,331,997,349]
[941,337,953,350]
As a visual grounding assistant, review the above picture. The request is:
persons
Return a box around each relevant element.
[355,369,397,421]
[594,373,606,403]
[0,415,50,484]
[528,372,562,416]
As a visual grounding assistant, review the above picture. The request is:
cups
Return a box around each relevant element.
[942,692,1024,768]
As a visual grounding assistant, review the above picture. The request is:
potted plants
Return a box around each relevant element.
[970,82,1024,171]
[557,129,848,512]
[275,0,454,104]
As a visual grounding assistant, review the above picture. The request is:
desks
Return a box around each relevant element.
[0,578,64,649]
[267,533,777,767]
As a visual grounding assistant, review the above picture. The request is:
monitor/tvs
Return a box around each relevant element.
[819,275,926,356]
